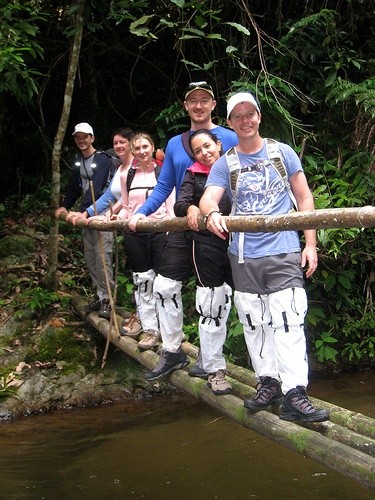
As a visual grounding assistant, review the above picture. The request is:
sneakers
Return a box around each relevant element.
[206,369,233,395]
[187,348,209,377]
[99,297,111,318]
[120,314,143,335]
[146,349,186,381]
[280,385,329,423]
[243,375,284,412]
[81,296,100,312]
[136,334,161,351]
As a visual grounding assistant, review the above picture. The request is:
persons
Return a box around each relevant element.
[54,122,185,350]
[128,82,238,381]
[199,93,331,423]
[174,129,235,395]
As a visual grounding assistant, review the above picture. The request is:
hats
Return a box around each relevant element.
[227,92,260,122]
[185,86,215,100]
[72,122,93,136]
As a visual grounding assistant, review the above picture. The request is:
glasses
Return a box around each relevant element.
[184,81,213,96]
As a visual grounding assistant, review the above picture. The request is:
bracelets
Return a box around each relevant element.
[204,211,221,231]
